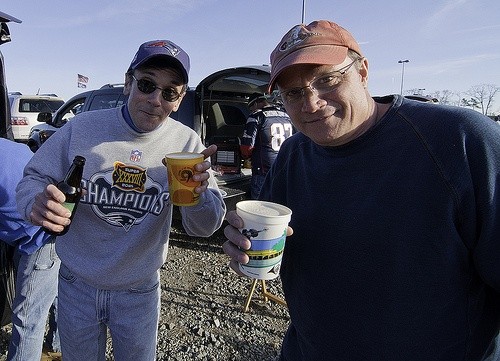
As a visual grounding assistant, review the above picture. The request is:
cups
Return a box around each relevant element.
[235,200,293,280]
[165,152,203,206]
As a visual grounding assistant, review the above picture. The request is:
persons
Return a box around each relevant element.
[222,20,500,361]
[0,137,62,361]
[238,91,298,201]
[16,40,227,361]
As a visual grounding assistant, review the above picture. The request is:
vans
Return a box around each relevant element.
[191,63,286,185]
[405,95,440,105]
[27,83,196,153]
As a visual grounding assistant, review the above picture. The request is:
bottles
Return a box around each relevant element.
[45,155,87,236]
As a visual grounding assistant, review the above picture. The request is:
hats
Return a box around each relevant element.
[268,20,362,96]
[247,93,266,108]
[130,39,190,84]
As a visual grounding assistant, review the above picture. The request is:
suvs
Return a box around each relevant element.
[7,91,76,144]
[0,10,23,142]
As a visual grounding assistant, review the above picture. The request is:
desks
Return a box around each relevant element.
[221,187,246,220]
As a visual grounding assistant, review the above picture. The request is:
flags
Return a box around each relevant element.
[78,74,88,83]
[78,83,86,88]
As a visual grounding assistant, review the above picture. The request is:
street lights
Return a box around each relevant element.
[398,59,410,96]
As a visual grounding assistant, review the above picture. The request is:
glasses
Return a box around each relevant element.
[131,74,182,102]
[276,57,364,103]
